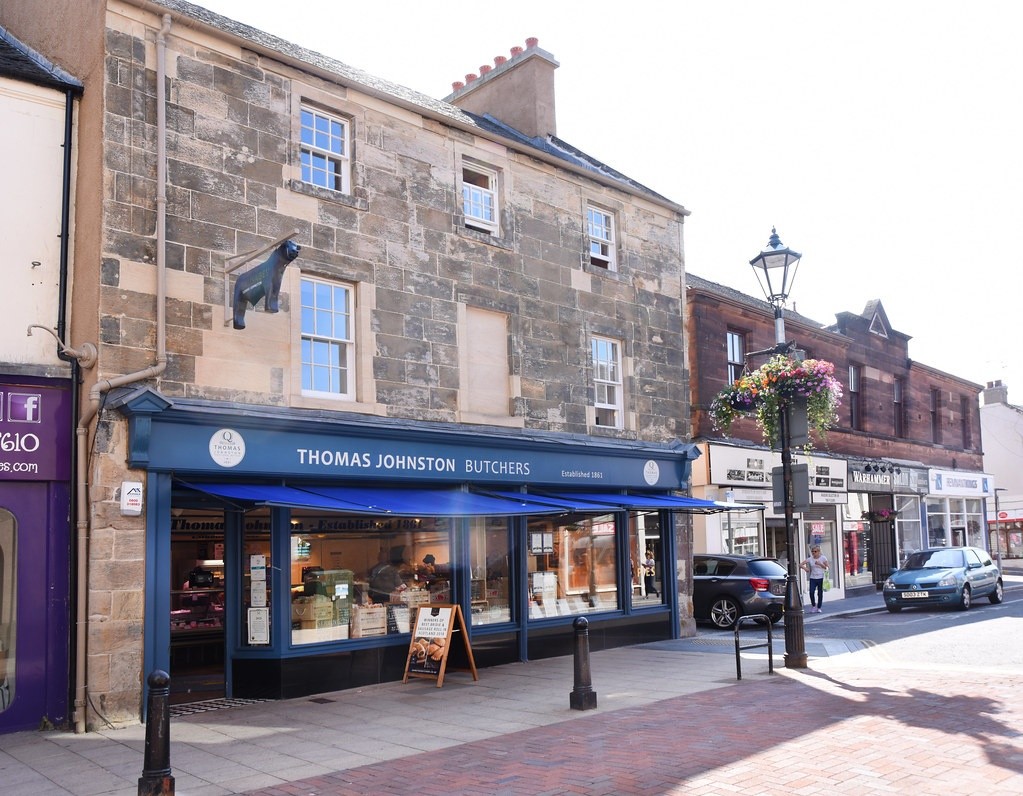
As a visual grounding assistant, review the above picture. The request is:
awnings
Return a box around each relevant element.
[184,482,767,519]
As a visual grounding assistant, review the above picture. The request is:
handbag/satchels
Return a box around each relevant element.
[646,566,655,577]
[823,567,830,592]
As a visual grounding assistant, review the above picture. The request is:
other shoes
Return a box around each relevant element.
[643,597,648,600]
[657,591,659,597]
[817,608,821,612]
[812,605,816,612]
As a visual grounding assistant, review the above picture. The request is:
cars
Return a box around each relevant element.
[882,546,1004,613]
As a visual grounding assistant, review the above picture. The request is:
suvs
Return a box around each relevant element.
[692,553,789,631]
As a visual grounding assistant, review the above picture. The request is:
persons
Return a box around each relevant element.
[641,551,659,600]
[799,546,829,613]
[368,551,402,604]
[183,566,213,597]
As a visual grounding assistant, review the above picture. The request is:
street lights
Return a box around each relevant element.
[749,223,810,670]
[994,487,1008,576]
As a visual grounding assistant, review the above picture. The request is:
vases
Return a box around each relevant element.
[780,384,798,400]
[730,399,755,413]
[873,515,896,522]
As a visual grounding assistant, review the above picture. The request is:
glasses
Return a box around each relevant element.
[811,550,819,553]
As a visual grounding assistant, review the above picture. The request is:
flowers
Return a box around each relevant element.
[707,356,842,449]
[860,508,899,519]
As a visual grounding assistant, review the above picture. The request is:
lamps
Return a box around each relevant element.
[860,460,901,475]
